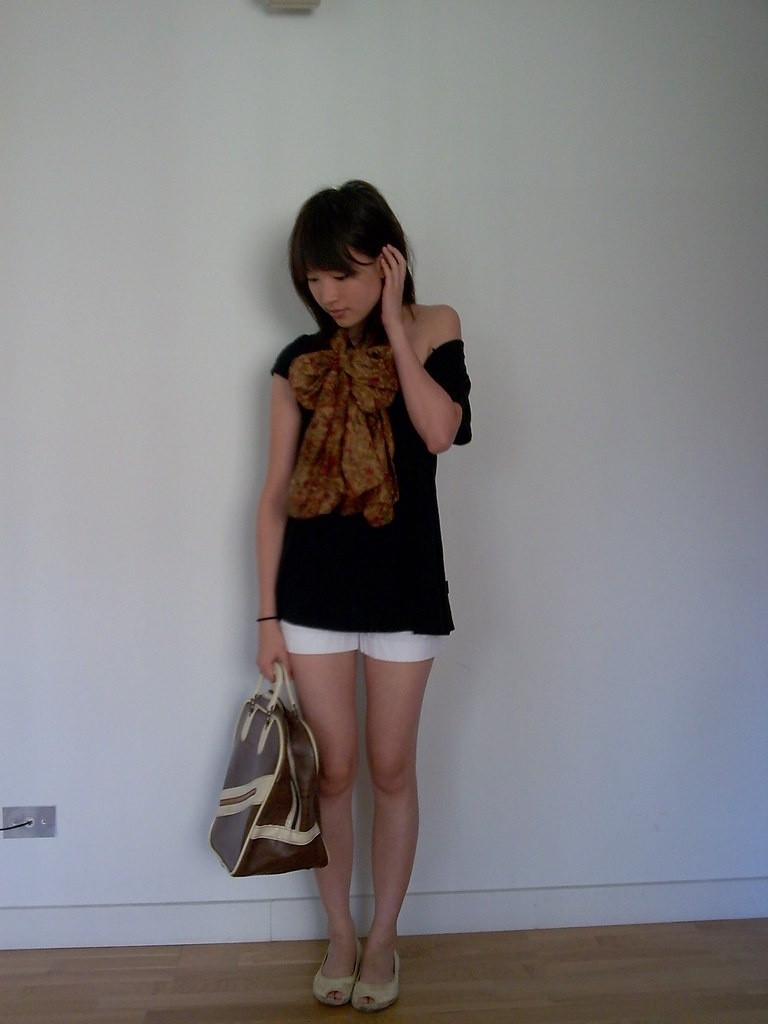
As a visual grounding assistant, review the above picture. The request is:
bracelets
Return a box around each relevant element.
[257,614,278,622]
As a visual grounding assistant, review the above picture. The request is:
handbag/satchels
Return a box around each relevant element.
[210,660,330,877]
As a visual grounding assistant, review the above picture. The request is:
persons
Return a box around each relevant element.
[207,180,473,1013]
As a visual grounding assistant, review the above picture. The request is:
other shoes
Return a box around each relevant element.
[352,951,401,1011]
[312,939,365,1006]
